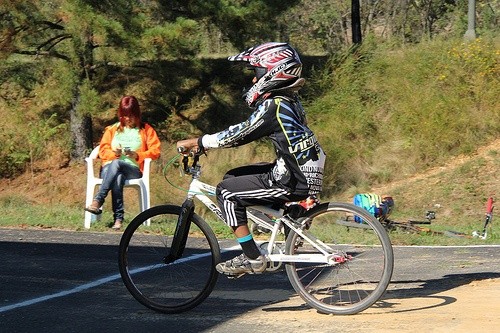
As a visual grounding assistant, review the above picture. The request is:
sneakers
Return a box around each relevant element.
[283,218,313,248]
[216,254,267,275]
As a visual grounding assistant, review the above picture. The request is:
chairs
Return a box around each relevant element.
[84,144,152,229]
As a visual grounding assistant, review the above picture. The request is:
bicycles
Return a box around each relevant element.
[118,145,395,315]
[335,194,495,240]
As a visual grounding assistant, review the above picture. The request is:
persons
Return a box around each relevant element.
[177,42,325,280]
[83,95,161,231]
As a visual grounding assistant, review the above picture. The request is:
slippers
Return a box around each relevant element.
[112,220,122,229]
[84,205,102,215]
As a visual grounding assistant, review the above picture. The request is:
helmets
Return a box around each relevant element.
[227,42,303,109]
[354,194,394,224]
